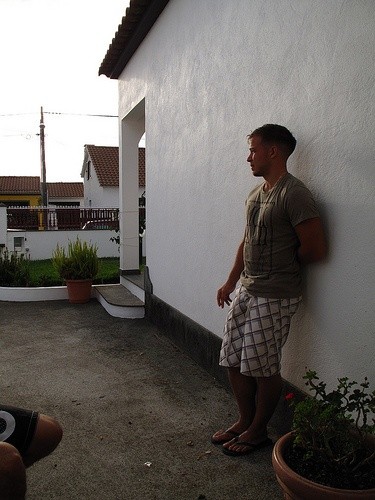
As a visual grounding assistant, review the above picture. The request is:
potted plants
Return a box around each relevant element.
[51,234,98,304]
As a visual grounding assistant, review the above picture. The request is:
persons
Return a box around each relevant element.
[0,404,63,500]
[210,123,328,457]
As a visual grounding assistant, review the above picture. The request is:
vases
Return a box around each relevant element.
[272,426,375,500]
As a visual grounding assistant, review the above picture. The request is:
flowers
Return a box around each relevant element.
[284,369,375,489]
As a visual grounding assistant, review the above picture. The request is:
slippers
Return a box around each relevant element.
[211,424,274,457]
